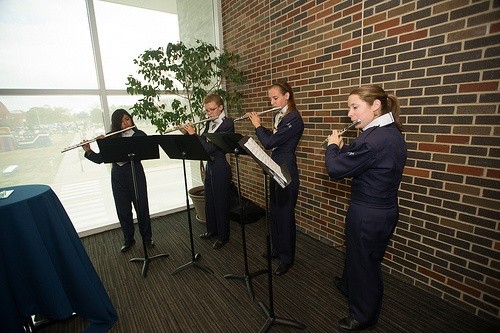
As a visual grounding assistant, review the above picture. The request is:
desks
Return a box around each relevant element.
[0,184,118,333]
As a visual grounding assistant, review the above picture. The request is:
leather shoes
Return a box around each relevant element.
[337,315,377,333]
[331,275,344,291]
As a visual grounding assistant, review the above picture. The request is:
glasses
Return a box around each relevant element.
[204,104,220,114]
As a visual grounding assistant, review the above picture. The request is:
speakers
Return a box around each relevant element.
[229,197,265,224]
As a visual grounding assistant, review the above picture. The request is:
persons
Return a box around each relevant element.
[80,109,154,252]
[325,84,407,331]
[178,95,236,249]
[245,81,304,275]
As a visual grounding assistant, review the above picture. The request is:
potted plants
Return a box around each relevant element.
[124,41,248,225]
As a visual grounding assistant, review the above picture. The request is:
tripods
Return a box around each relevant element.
[149,136,214,275]
[238,135,306,333]
[97,137,168,274]
[204,132,268,302]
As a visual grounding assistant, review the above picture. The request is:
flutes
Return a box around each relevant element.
[321,119,360,150]
[164,117,219,133]
[60,127,136,153]
[234,107,281,122]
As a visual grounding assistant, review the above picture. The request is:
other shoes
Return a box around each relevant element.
[144,238,155,249]
[273,259,294,276]
[199,232,217,239]
[212,238,228,249]
[261,251,279,259]
[121,240,136,252]
